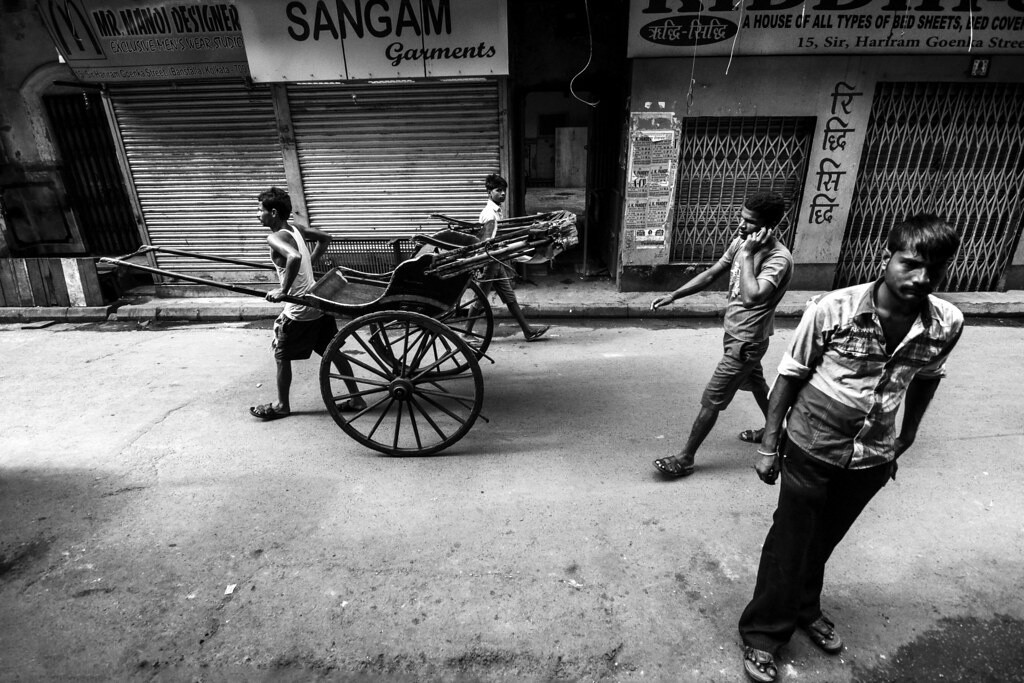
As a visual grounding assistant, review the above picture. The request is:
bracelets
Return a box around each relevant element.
[756,448,778,457]
[667,292,675,303]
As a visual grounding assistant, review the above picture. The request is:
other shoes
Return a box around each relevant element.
[808,615,843,655]
[743,646,778,683]
[524,325,550,341]
[460,339,484,347]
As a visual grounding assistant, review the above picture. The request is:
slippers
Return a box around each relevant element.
[652,454,695,478]
[249,403,291,418]
[336,400,364,412]
[739,427,782,445]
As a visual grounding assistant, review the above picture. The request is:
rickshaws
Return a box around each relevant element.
[98,210,580,457]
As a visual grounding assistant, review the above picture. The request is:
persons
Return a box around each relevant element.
[645,188,795,478]
[248,186,368,420]
[736,211,965,683]
[455,172,551,345]
